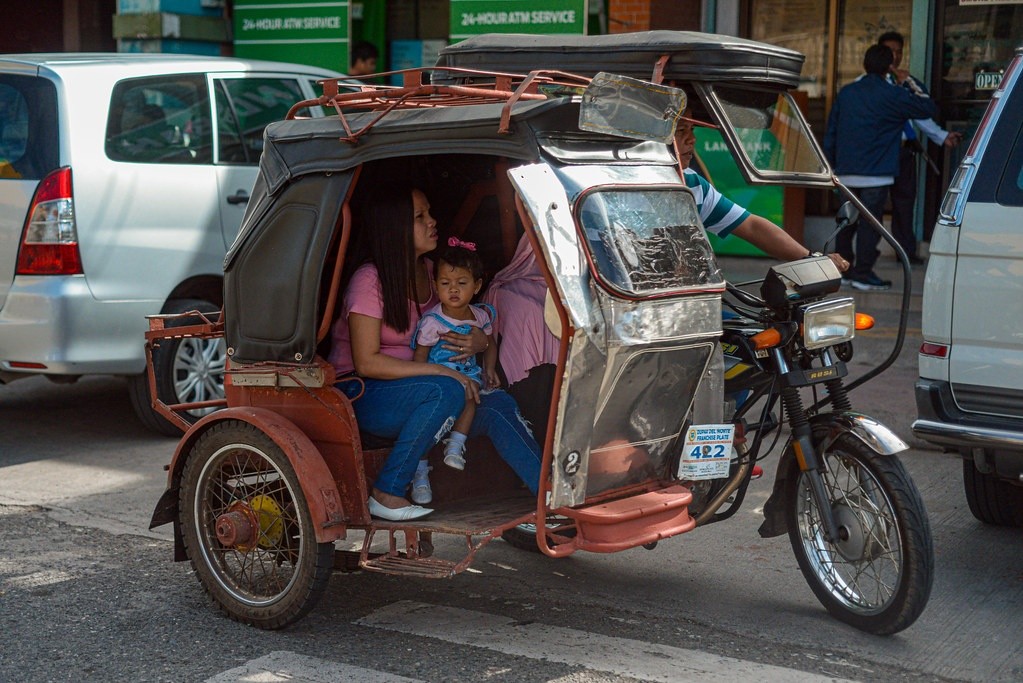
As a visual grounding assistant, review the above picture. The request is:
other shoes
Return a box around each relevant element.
[852,272,891,291]
[367,496,435,523]
[411,476,432,504]
[751,466,764,480]
[442,445,468,471]
[841,274,852,285]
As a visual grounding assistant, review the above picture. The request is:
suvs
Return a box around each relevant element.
[909,44,1023,523]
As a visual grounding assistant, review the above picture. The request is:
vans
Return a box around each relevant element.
[0,55,402,436]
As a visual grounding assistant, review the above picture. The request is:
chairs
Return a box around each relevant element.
[123,105,167,146]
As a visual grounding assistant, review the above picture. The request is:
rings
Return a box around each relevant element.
[460,347,463,352]
[843,260,846,262]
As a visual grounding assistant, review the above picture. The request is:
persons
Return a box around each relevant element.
[410,236,502,504]
[112,88,166,149]
[580,108,850,480]
[346,40,379,85]
[823,31,965,290]
[328,171,551,522]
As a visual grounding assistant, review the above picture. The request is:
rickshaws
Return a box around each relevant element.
[141,29,936,639]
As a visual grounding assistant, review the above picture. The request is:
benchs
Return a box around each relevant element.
[367,416,549,505]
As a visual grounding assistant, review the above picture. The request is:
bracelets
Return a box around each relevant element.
[808,248,812,256]
[481,341,489,352]
